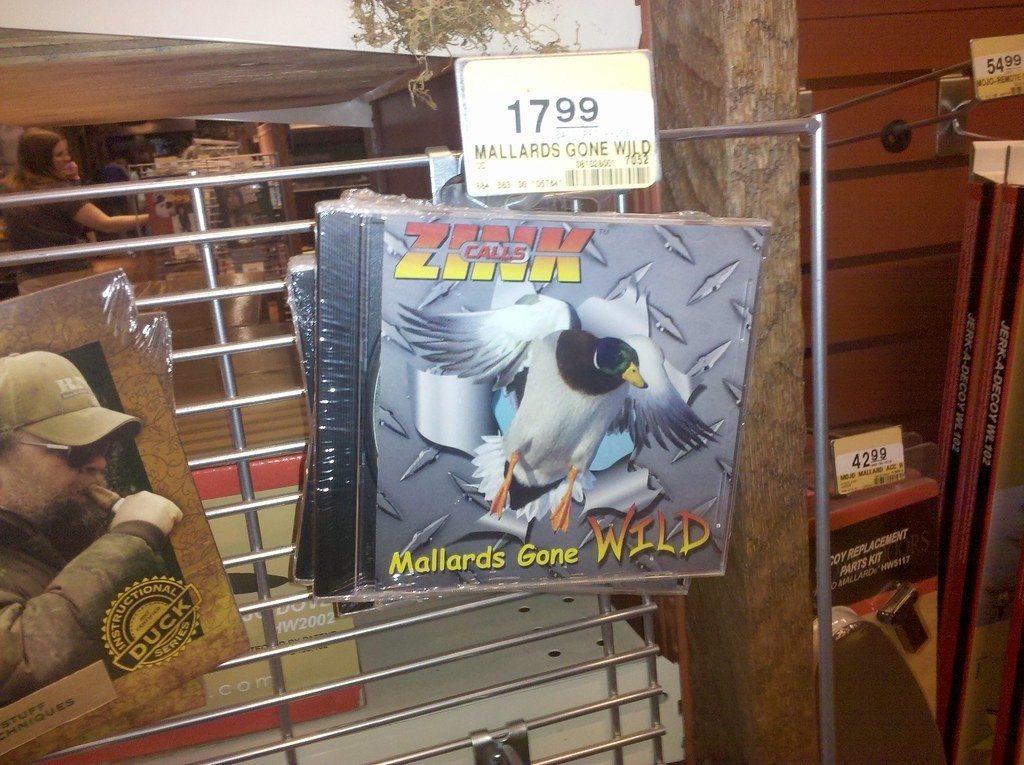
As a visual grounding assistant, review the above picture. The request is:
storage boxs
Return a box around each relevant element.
[805,421,908,496]
[0,325,364,765]
[813,574,939,723]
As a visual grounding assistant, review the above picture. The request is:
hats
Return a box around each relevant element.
[0,351,143,447]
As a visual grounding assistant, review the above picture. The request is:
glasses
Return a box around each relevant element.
[16,438,113,468]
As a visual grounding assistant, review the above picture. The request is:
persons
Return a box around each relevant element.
[95,149,139,241]
[218,184,252,248]
[0,350,185,706]
[177,207,192,232]
[0,127,150,274]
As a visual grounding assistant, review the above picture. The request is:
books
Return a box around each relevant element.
[143,173,203,264]
[933,141,1024,765]
[201,162,290,269]
[0,269,250,765]
[284,191,766,597]
